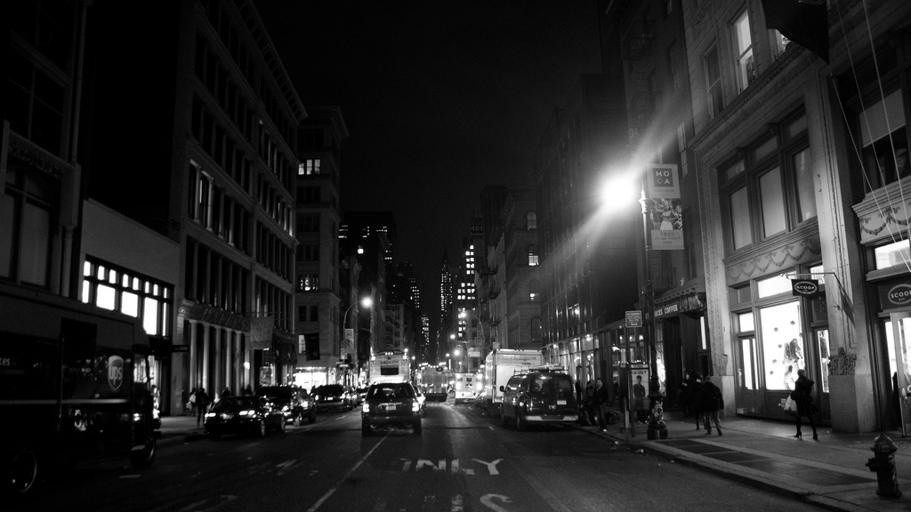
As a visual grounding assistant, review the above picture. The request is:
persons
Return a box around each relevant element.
[575,376,618,433]
[699,376,725,437]
[790,369,818,442]
[651,376,660,391]
[634,375,645,398]
[635,410,648,425]
[682,372,707,430]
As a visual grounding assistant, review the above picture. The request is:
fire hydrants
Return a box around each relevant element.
[864,432,903,500]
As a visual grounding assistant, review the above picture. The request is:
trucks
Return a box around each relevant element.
[1,286,164,498]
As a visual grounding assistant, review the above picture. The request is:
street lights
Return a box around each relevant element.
[598,165,667,439]
[342,297,373,329]
[457,311,486,342]
[441,331,472,375]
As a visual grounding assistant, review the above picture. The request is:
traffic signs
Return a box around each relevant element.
[624,310,641,328]
